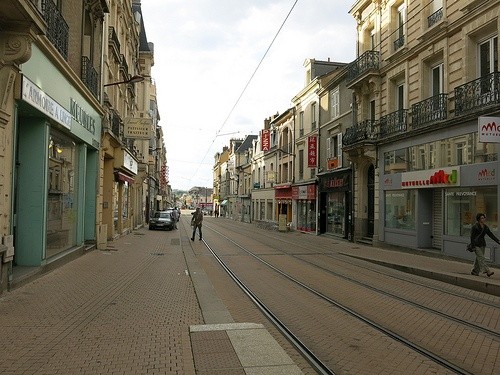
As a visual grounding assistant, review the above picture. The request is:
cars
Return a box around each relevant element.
[147,205,181,231]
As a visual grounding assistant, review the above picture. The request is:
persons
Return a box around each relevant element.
[173,207,178,230]
[470,213,500,277]
[178,208,181,222]
[214,209,218,218]
[191,207,203,241]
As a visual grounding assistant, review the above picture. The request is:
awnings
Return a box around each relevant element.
[221,200,228,205]
